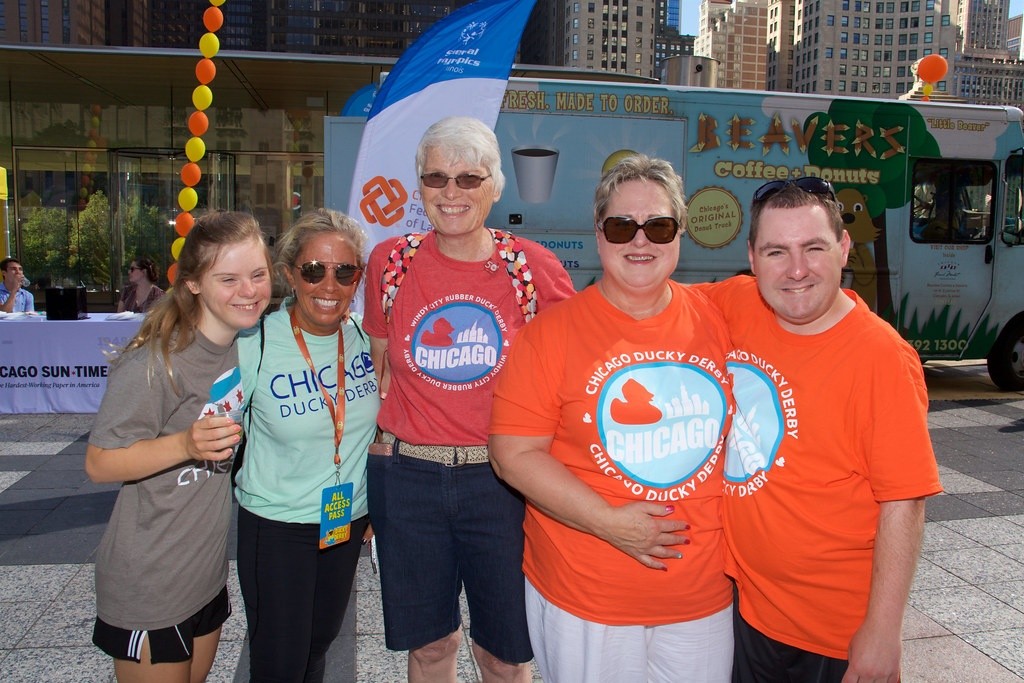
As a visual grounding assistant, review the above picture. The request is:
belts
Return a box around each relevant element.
[382,432,490,467]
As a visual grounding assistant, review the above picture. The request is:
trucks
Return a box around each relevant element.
[322,69,1024,397]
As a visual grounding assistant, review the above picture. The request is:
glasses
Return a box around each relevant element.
[129,266,144,271]
[420,172,491,189]
[753,176,836,203]
[291,261,363,286]
[597,216,681,244]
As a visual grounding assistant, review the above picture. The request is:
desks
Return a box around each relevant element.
[0,313,219,414]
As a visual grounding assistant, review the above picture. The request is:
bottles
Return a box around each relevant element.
[16,274,30,288]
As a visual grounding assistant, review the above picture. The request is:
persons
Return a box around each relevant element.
[920,188,970,240]
[118,256,166,313]
[487,153,733,683]
[239,209,380,683]
[362,119,577,683]
[689,174,942,683]
[83,212,272,683]
[0,258,34,313]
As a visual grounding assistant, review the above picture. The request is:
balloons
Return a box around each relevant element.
[77,104,101,210]
[918,54,948,101]
[167,0,227,288]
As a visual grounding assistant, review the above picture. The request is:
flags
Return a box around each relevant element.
[345,0,533,315]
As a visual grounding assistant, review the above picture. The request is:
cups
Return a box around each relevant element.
[511,143,560,204]
[209,410,244,446]
